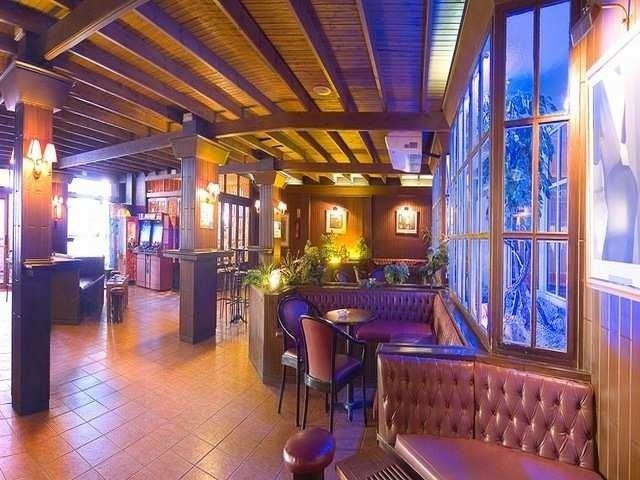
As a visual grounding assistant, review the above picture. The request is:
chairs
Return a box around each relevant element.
[276,293,341,427]
[297,311,369,433]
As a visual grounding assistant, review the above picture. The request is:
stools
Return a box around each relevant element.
[216,263,252,328]
[105,270,131,325]
[282,426,336,480]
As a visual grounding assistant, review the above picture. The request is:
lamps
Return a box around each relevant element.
[204,182,222,207]
[27,137,57,180]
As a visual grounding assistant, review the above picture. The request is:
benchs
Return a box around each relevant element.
[287,283,446,343]
[374,340,605,480]
[50,256,106,324]
[386,289,490,355]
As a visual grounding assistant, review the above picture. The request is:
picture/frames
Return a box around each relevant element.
[394,208,418,235]
[323,208,350,237]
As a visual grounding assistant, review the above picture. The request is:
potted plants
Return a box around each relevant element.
[241,239,445,294]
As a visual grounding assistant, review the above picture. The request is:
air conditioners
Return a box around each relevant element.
[384,129,423,174]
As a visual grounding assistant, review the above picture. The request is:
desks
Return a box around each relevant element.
[323,306,379,415]
